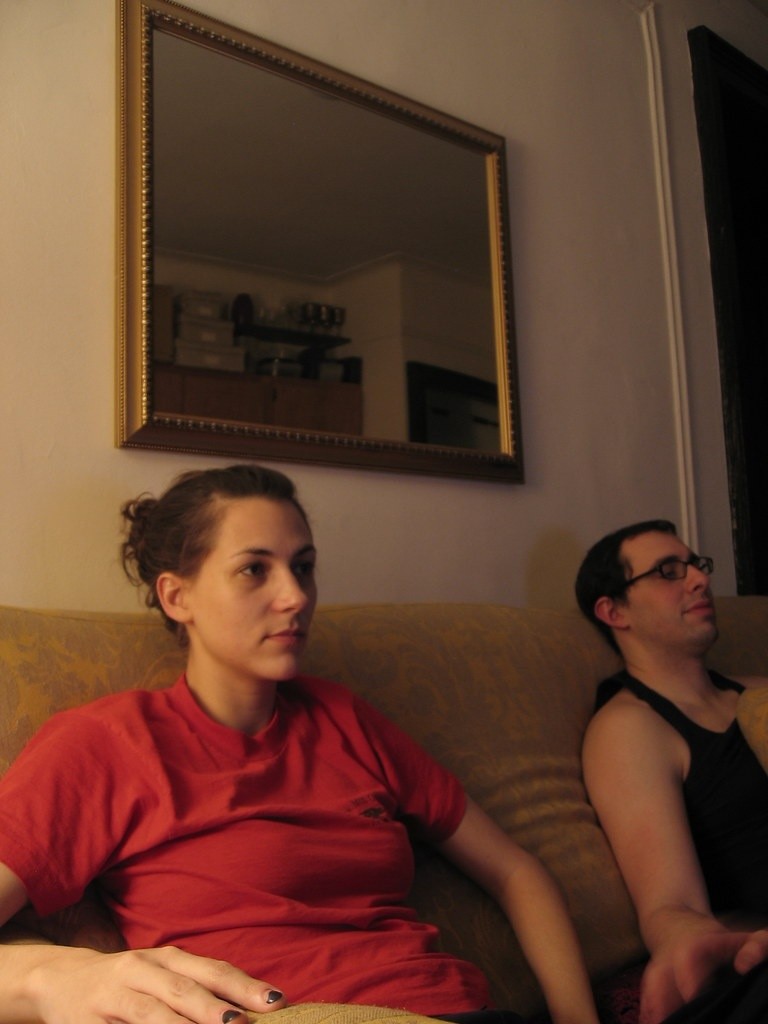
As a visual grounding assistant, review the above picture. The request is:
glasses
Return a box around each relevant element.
[627,554,714,585]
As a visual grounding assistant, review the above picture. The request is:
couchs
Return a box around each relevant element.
[0,597,768,1024]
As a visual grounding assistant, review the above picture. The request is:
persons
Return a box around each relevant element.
[0,463,600,1024]
[574,518,768,1023]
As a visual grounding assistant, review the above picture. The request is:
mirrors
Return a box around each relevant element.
[113,0,529,486]
